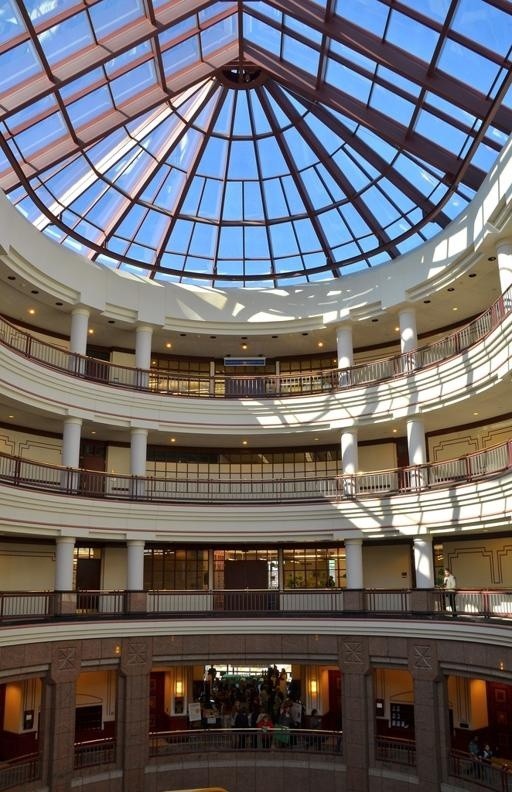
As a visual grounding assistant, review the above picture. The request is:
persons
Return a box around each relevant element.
[463,734,481,780]
[325,576,335,588]
[204,664,330,754]
[479,742,495,784]
[437,567,458,618]
